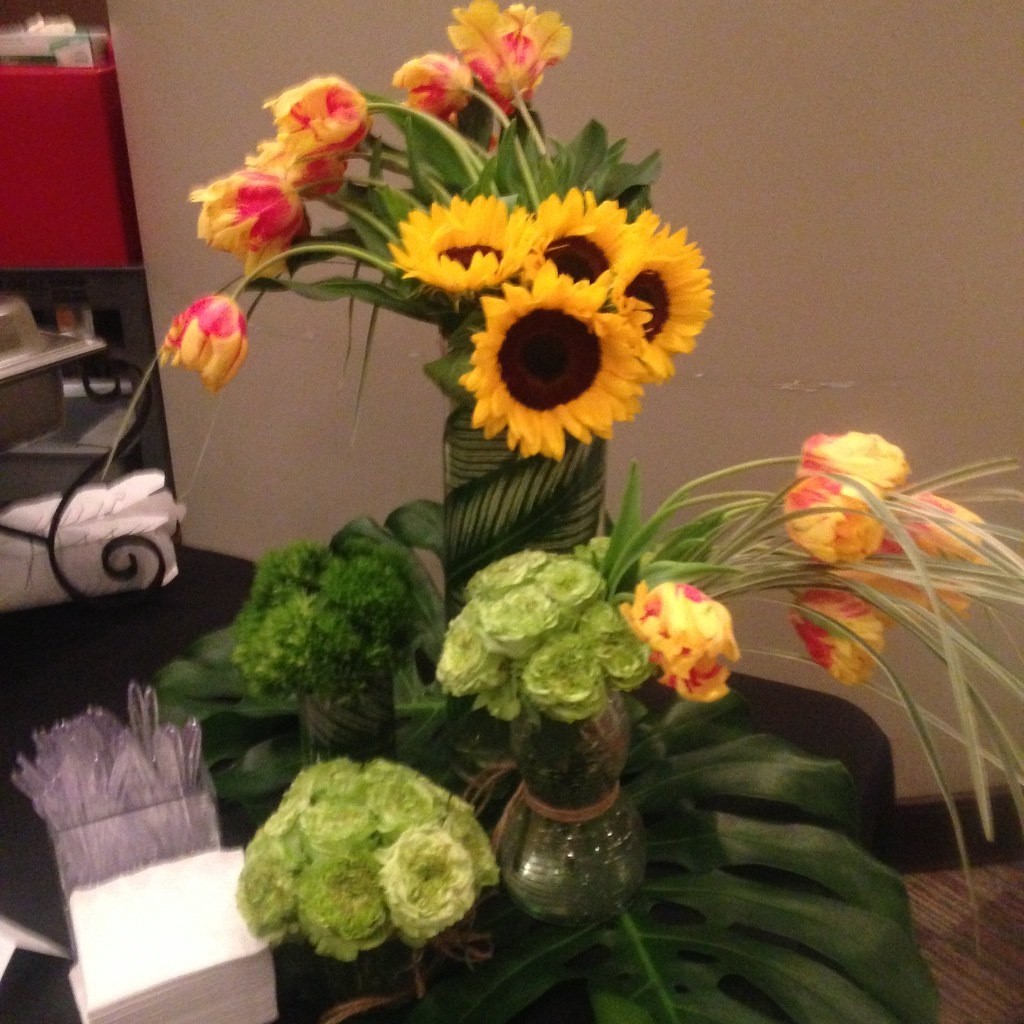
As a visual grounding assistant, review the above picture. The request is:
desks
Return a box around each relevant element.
[0,551,895,1024]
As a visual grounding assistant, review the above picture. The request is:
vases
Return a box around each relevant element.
[302,938,421,1016]
[496,690,645,931]
[443,411,607,645]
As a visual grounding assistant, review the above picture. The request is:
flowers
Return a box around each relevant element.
[96,0,1024,1024]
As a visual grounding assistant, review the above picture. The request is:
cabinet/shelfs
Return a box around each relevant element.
[0,0,176,611]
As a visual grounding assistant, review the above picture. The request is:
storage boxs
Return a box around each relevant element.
[0,40,126,266]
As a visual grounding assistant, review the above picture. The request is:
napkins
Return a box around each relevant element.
[66,848,287,1024]
